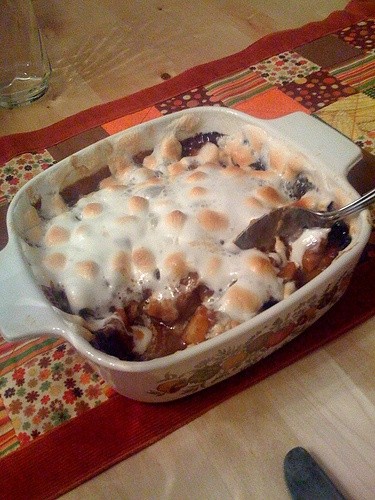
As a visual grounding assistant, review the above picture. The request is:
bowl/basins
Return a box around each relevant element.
[1,105,373,404]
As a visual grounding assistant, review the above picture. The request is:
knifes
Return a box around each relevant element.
[282,444,346,500]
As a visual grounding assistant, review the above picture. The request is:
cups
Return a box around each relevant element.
[0,0,52,109]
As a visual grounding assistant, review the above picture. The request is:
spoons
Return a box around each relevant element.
[232,189,375,260]
